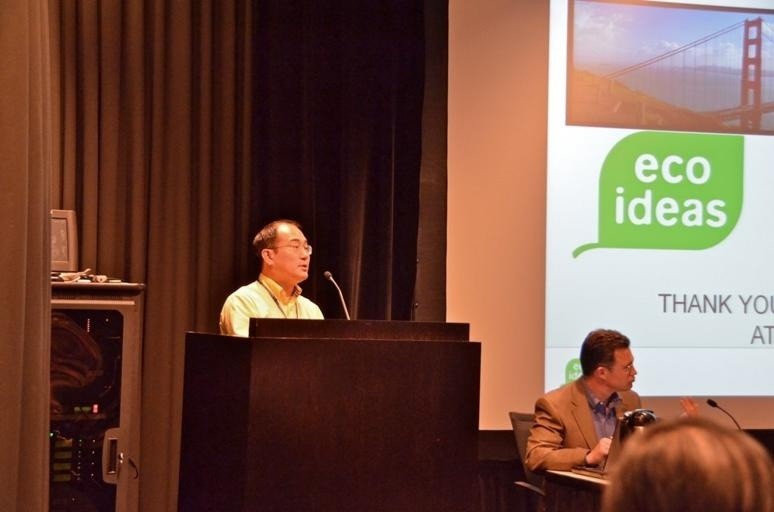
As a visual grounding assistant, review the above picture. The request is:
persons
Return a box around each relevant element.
[522,328,698,512]
[600,415,774,511]
[219,220,326,337]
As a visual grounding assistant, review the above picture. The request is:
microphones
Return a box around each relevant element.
[324,271,350,320]
[707,399,741,431]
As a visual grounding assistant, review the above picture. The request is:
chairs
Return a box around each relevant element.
[509,412,546,512]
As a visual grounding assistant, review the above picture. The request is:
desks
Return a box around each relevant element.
[545,469,611,512]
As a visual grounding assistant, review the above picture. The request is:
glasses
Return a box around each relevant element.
[276,244,314,256]
[604,364,634,372]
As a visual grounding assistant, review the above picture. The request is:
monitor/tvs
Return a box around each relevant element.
[51,209,78,282]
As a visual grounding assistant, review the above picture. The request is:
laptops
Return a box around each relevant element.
[570,417,652,481]
